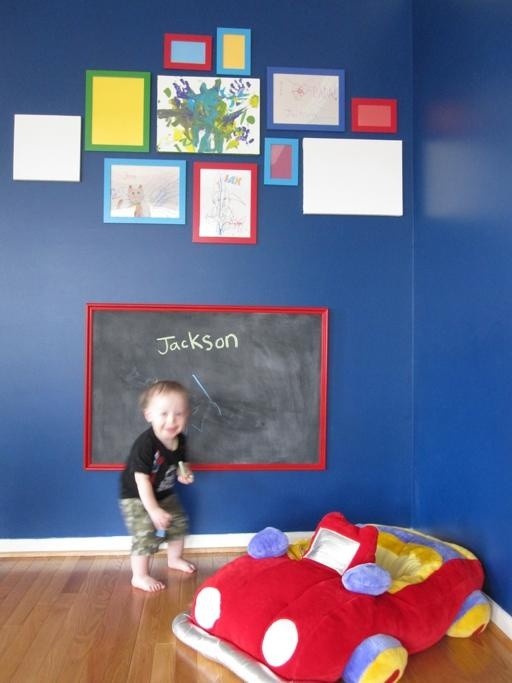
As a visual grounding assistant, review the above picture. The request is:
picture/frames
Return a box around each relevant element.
[263,137,299,186]
[84,69,150,153]
[216,27,251,76]
[103,157,186,226]
[352,97,397,133]
[266,66,346,132]
[164,32,212,72]
[192,161,258,244]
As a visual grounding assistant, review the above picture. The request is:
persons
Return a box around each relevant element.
[119,379,195,592]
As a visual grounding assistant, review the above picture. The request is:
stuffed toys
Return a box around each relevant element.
[171,511,491,682]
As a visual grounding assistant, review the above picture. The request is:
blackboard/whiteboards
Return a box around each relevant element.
[83,303,329,471]
